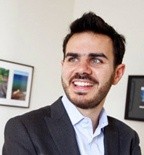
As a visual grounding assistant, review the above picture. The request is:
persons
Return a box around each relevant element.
[2,11,141,155]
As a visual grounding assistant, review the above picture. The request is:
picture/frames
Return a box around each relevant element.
[124,74,144,122]
[0,59,34,109]
